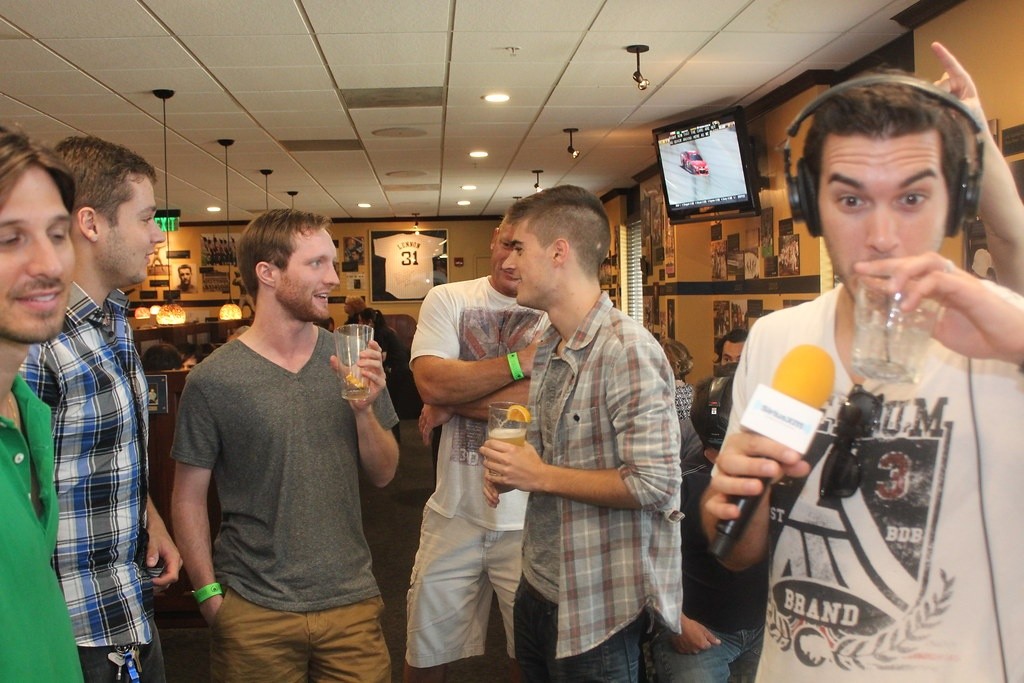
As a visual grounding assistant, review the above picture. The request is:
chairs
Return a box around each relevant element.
[381,313,416,347]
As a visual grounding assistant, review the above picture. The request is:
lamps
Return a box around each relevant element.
[155,89,186,325]
[625,45,652,92]
[531,169,544,193]
[411,212,421,237]
[217,139,243,321]
[563,128,581,158]
[133,280,150,319]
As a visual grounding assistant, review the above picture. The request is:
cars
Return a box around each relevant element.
[680,151,709,174]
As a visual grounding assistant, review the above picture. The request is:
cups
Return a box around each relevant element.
[333,324,374,400]
[851,275,948,384]
[488,401,531,464]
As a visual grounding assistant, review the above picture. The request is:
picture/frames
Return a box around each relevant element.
[369,229,450,305]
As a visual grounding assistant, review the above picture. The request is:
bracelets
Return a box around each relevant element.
[507,352,524,381]
[193,582,223,604]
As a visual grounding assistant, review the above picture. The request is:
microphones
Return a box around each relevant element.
[708,344,838,560]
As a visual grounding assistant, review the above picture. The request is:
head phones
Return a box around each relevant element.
[783,75,987,239]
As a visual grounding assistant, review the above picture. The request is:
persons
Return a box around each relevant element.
[699,71,1024,683]
[201,234,237,267]
[657,338,696,422]
[169,209,400,683]
[480,186,683,683]
[141,342,202,371]
[149,245,166,272]
[643,362,808,683]
[232,274,255,317]
[344,296,409,385]
[176,264,197,294]
[344,238,363,262]
[931,43,1024,298]
[403,199,552,683]
[715,330,749,366]
[0,125,85,683]
[20,134,185,683]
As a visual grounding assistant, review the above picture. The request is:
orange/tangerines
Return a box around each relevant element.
[507,405,530,422]
[346,374,364,388]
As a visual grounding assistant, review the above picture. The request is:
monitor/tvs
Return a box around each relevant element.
[652,104,767,224]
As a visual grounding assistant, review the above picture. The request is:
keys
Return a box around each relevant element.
[108,646,142,683]
